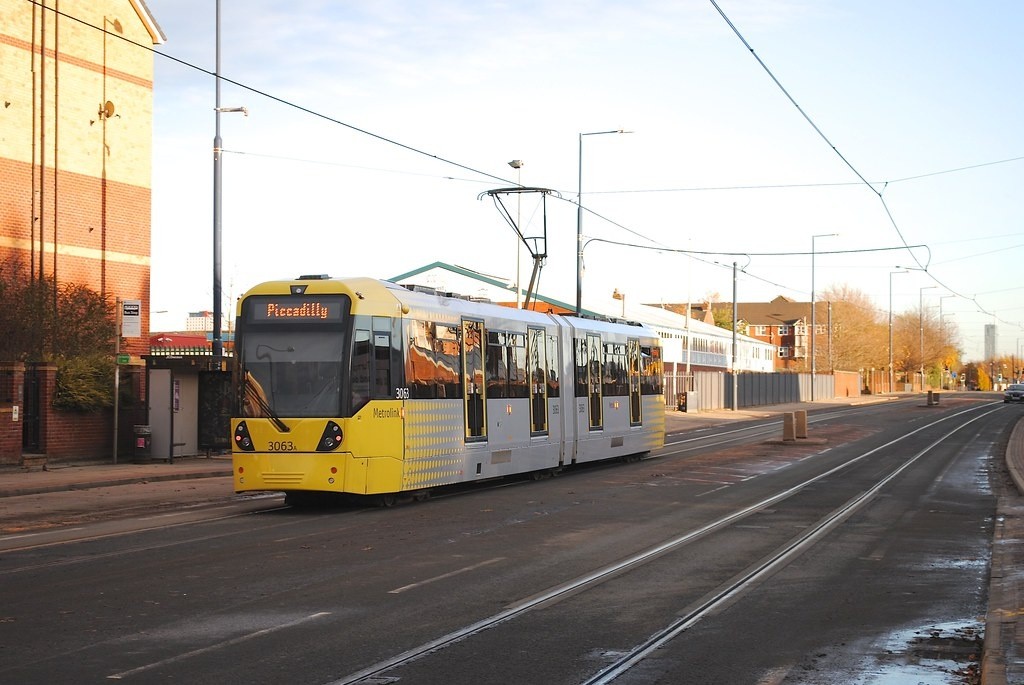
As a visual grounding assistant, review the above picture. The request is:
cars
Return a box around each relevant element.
[1003,384,1024,402]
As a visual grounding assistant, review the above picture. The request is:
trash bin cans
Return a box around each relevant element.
[132,424,151,464]
[686,391,698,413]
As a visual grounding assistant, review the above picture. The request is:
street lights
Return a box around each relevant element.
[920,286,937,390]
[940,295,957,389]
[508,159,526,310]
[810,233,841,401]
[574,129,637,318]
[889,270,909,393]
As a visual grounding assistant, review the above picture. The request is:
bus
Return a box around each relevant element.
[228,187,666,509]
